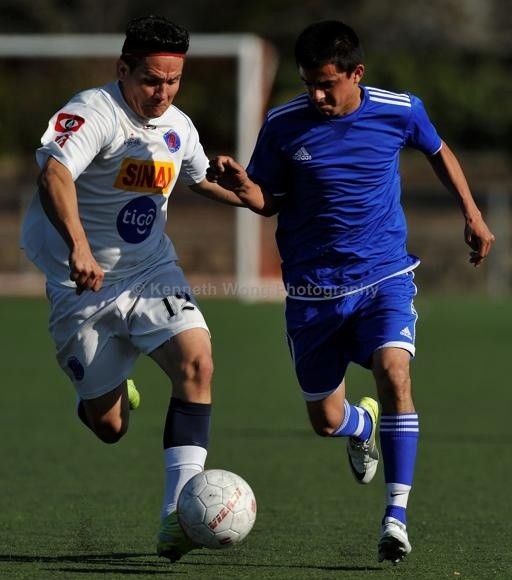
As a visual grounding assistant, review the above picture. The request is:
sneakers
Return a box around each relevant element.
[126,378,140,410]
[156,509,200,562]
[346,396,380,485]
[378,516,412,565]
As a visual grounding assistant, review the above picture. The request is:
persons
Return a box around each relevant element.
[205,20,495,560]
[18,12,246,565]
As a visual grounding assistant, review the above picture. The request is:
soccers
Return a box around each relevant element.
[177,470,256,548]
